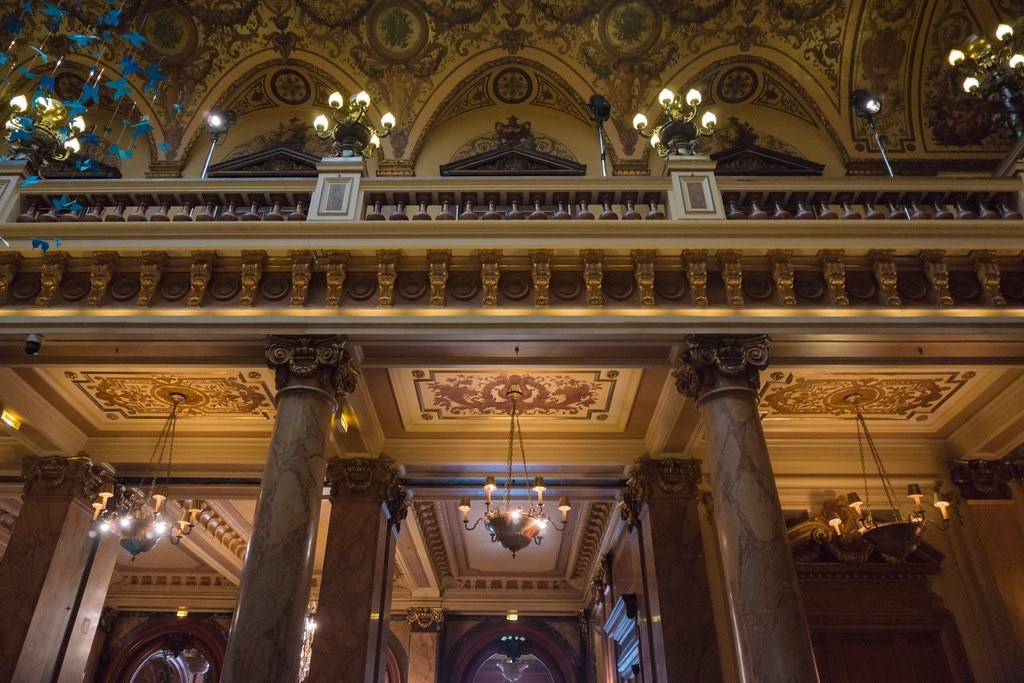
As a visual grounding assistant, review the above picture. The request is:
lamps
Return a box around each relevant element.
[200,109,236,179]
[848,88,911,220]
[829,394,951,560]
[314,90,395,158]
[184,651,210,677]
[91,393,201,561]
[586,93,611,178]
[948,24,1024,140]
[4,95,87,171]
[633,88,716,157]
[496,640,529,683]
[459,383,572,558]
